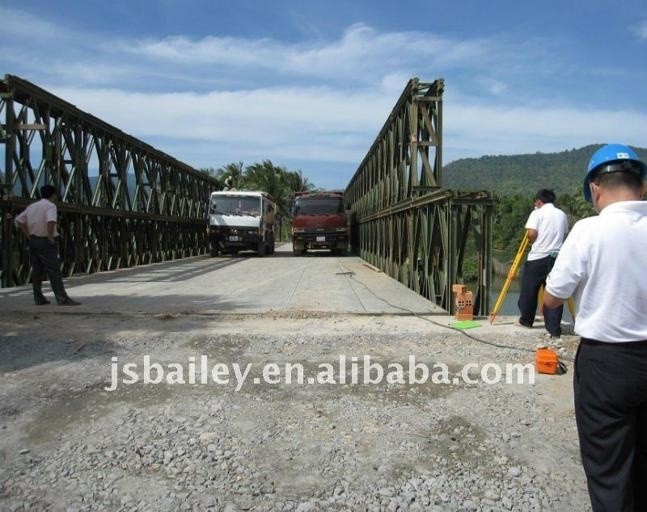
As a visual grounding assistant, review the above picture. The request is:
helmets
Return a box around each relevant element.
[582,142,646,201]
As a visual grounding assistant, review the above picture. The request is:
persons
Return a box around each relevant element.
[542,143,646,512]
[14,184,81,307]
[518,188,570,340]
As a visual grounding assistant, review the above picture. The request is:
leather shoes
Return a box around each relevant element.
[36,299,52,306]
[59,297,82,306]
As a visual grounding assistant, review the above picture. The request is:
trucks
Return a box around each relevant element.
[204,189,277,258]
[289,190,352,258]
